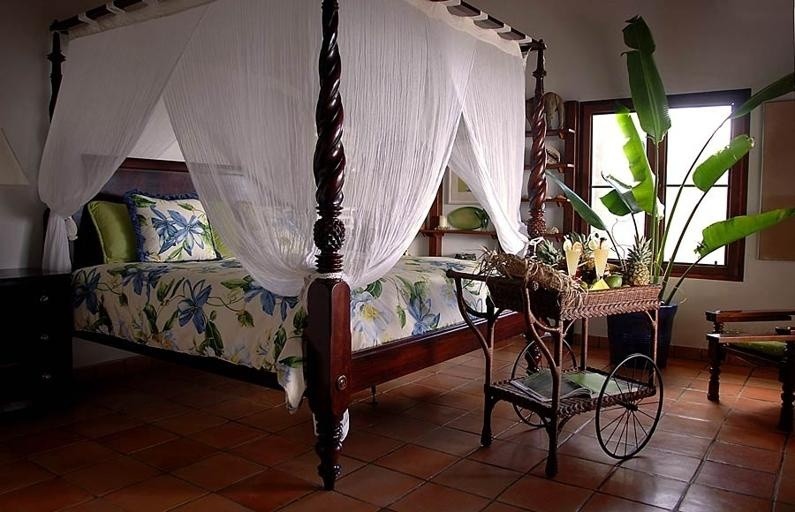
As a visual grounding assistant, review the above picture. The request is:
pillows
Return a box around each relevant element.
[206,201,302,260]
[89,201,140,264]
[124,190,221,262]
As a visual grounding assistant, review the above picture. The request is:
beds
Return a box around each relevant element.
[40,0,547,489]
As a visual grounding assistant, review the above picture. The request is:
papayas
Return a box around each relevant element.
[606,274,623,289]
[446,207,489,229]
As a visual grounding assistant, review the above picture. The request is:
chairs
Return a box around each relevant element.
[704,308,795,433]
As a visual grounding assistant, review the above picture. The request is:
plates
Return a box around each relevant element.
[447,206,483,229]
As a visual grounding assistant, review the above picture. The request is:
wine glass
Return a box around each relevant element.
[594,250,608,281]
[565,250,581,279]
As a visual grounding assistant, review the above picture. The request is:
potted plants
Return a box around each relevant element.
[543,15,795,370]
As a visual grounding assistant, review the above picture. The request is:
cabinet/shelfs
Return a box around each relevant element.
[1,268,73,419]
[419,101,578,262]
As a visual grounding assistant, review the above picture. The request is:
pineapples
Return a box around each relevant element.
[532,237,569,271]
[566,232,595,274]
[628,234,653,287]
